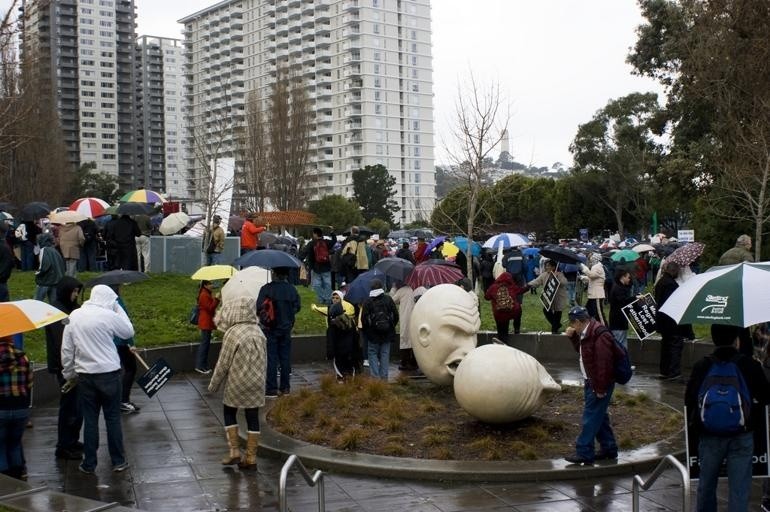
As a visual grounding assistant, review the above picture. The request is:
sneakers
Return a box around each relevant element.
[565,455,593,465]
[193,360,419,398]
[5,401,141,478]
[594,451,617,460]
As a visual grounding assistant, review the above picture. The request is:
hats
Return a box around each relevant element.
[568,306,590,321]
[330,302,343,314]
[332,290,343,300]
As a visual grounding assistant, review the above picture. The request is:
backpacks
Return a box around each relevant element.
[592,325,632,385]
[14,224,28,241]
[697,355,753,431]
[496,285,514,311]
[362,299,394,342]
[314,240,329,263]
[202,226,219,254]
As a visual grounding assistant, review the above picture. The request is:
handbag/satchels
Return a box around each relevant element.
[190,306,199,325]
[341,253,356,277]
[602,264,614,290]
[259,296,275,326]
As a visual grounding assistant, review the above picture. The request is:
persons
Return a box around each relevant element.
[562,306,618,464]
[208,296,267,468]
[1,189,769,396]
[1,277,140,484]
[684,324,769,511]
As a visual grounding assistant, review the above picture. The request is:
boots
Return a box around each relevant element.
[223,424,242,465]
[238,430,261,472]
